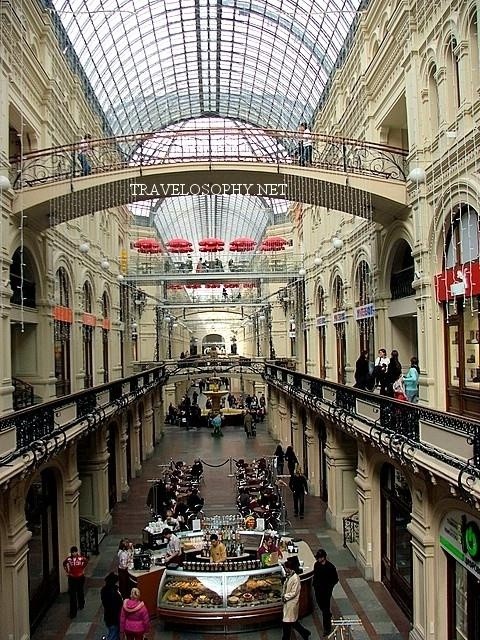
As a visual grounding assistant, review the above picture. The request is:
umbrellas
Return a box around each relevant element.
[133,235,289,273]
[166,282,257,303]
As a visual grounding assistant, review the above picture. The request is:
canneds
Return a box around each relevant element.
[183,559,260,572]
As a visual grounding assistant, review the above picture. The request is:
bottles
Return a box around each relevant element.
[183,559,261,572]
[202,514,244,542]
[275,539,298,553]
[226,543,244,557]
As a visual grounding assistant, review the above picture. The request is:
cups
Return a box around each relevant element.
[134,549,140,555]
[145,517,173,534]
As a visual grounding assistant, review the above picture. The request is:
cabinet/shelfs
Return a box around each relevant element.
[443,294,480,423]
[155,565,287,635]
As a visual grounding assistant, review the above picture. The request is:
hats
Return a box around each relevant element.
[105,572,119,585]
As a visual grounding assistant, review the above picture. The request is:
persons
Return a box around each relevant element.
[256,535,283,563]
[353,349,369,391]
[279,561,312,640]
[163,376,266,439]
[146,456,281,531]
[117,537,128,557]
[119,540,135,599]
[311,548,339,637]
[298,122,315,167]
[400,356,420,404]
[207,534,227,563]
[380,350,402,397]
[99,572,124,640]
[289,470,309,519]
[76,132,95,175]
[274,444,285,475]
[283,446,299,475]
[161,528,183,565]
[62,546,89,619]
[374,348,390,391]
[119,587,151,640]
